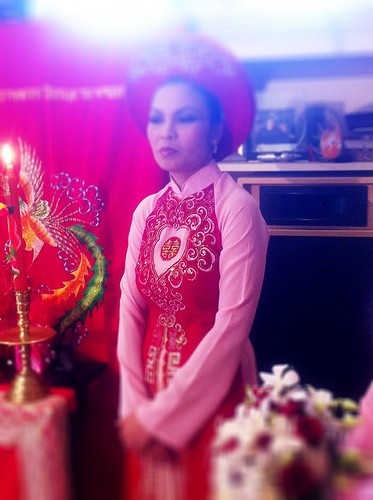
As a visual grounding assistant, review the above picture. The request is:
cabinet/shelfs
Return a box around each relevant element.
[214,160,373,238]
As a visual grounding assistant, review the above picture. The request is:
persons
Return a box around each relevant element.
[117,31,270,499]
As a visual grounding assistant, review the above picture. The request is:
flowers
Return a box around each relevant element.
[243,363,361,478]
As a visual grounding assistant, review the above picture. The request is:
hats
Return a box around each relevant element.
[126,28,257,163]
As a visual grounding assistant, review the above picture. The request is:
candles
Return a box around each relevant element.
[0,142,29,293]
